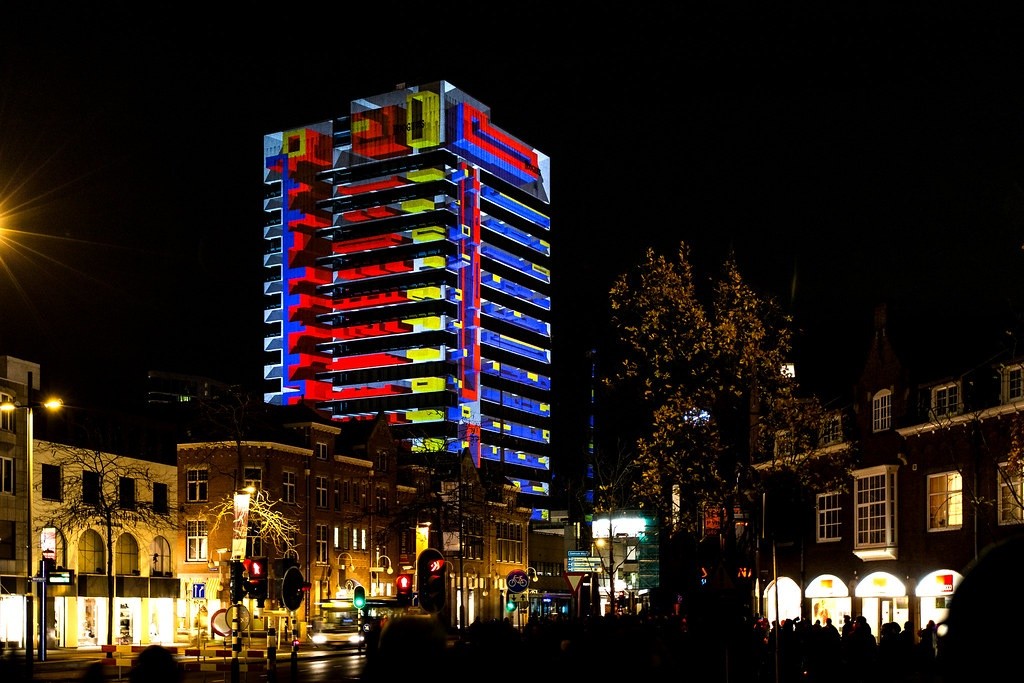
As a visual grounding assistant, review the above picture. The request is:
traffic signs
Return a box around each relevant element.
[567,550,605,573]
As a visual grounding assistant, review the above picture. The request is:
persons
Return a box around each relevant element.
[932,535,1024,683]
[353,610,935,683]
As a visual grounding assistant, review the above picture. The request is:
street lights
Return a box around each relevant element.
[1,396,64,660]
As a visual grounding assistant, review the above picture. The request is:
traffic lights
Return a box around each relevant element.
[396,574,413,607]
[417,548,447,614]
[244,557,269,600]
[282,566,312,611]
[353,586,366,610]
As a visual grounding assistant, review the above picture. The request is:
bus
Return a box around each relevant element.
[361,600,418,634]
